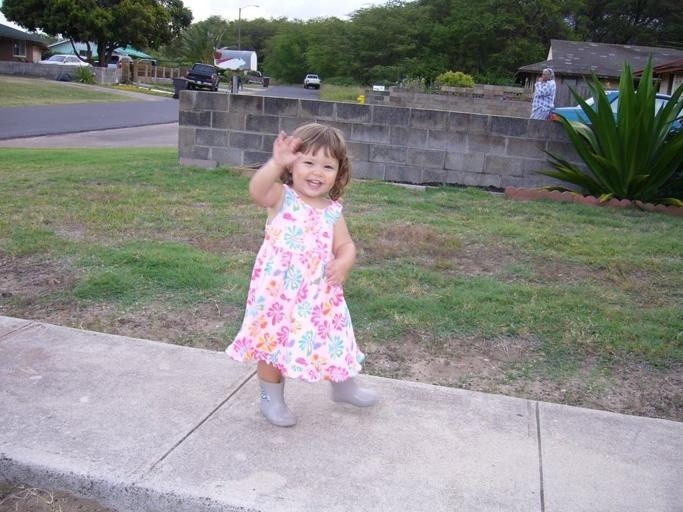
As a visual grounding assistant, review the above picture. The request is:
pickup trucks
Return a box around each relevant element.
[303,73,320,90]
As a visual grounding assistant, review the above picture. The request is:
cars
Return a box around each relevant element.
[548,89,683,134]
[136,58,159,66]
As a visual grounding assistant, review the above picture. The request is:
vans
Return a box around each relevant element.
[106,53,134,68]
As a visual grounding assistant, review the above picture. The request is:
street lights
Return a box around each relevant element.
[237,4,260,49]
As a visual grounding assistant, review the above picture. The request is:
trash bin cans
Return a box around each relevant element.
[172,77,188,98]
[262,77,270,87]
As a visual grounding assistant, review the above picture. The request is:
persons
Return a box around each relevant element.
[530,68,556,120]
[226,124,378,426]
[225,70,243,93]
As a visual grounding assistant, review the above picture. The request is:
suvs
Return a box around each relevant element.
[187,62,222,93]
[36,54,92,67]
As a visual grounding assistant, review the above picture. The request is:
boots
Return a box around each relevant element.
[258,377,298,428]
[330,378,376,408]
